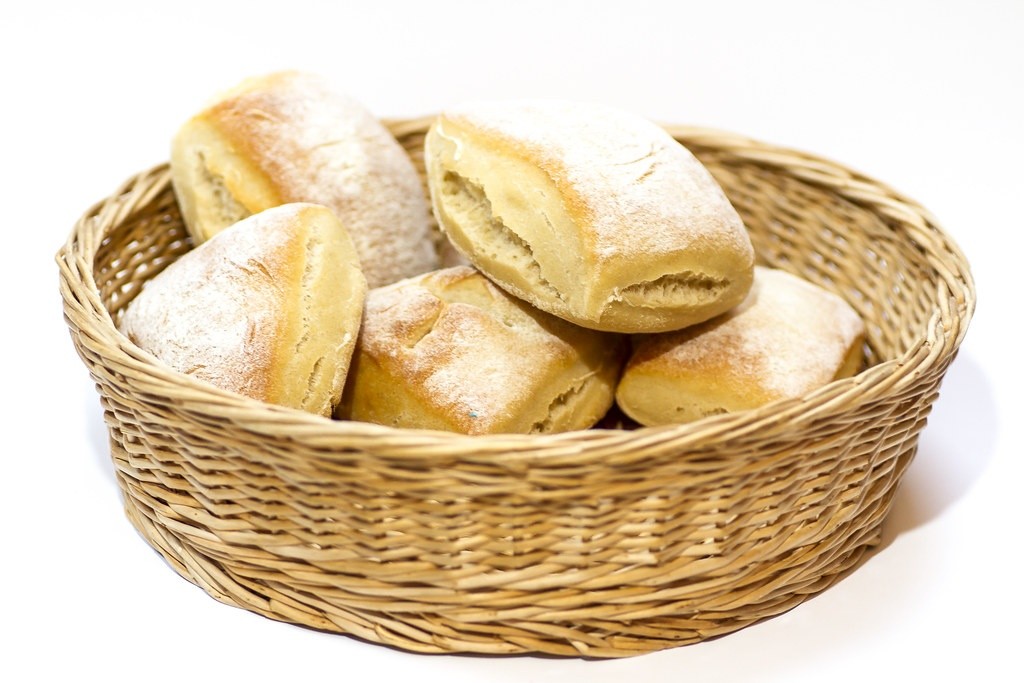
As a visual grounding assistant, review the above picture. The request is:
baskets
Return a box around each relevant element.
[51,107,976,659]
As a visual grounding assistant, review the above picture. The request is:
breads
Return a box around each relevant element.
[116,64,868,436]
